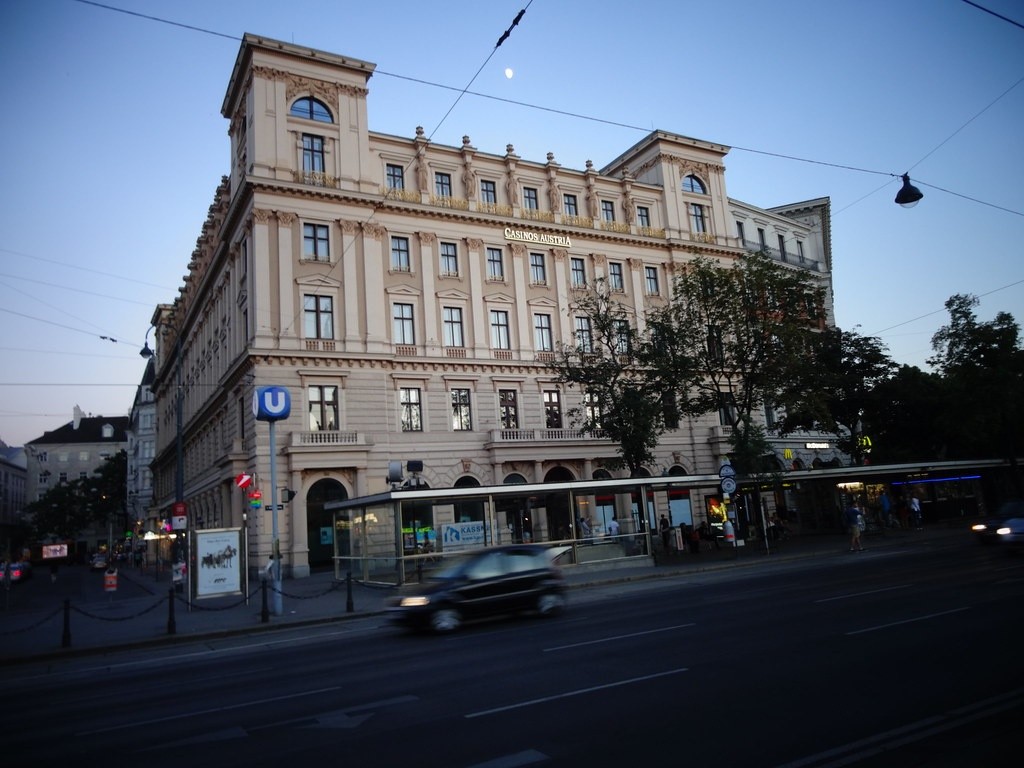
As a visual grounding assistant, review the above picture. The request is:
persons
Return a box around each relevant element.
[846,490,923,552]
[575,517,592,543]
[49,560,60,583]
[659,514,750,551]
[90,548,144,569]
[609,516,621,542]
[772,512,793,542]
[421,537,435,565]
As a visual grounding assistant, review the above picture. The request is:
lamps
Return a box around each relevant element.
[281,489,295,503]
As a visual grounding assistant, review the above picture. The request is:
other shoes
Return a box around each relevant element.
[849,547,855,551]
[859,547,864,551]
[920,528,923,530]
[917,528,919,531]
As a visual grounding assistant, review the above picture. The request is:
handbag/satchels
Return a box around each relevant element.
[915,514,921,518]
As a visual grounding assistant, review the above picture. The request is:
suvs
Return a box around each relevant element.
[384,544,566,637]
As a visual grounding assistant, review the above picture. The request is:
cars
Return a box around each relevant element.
[970,497,1024,544]
[0,560,32,584]
